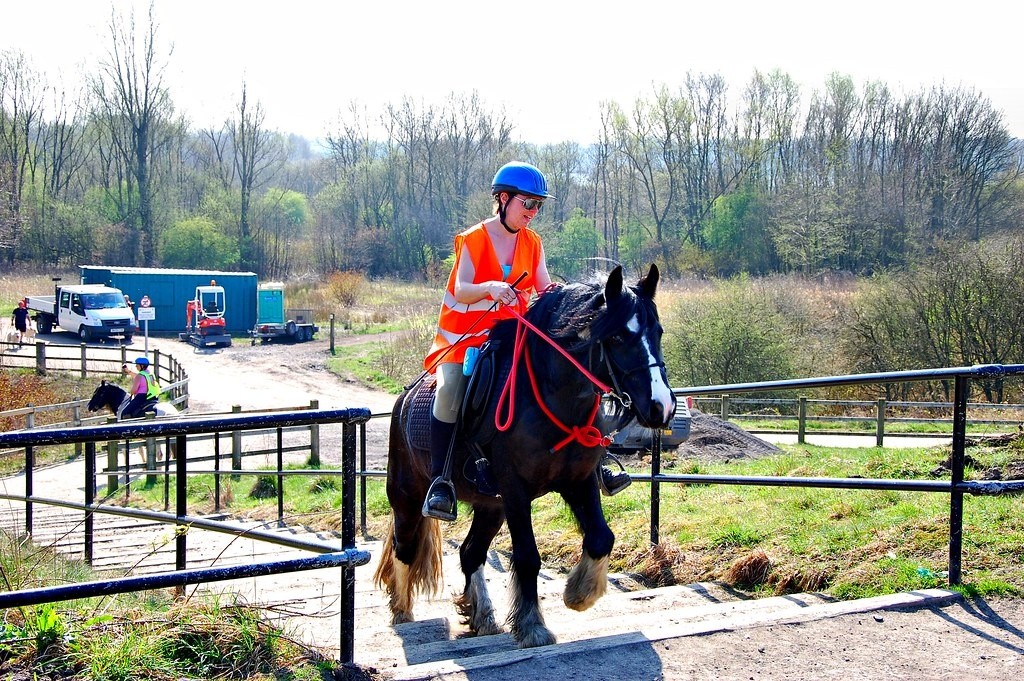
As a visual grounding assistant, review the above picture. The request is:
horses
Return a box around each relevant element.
[372,256,676,648]
[87,379,180,472]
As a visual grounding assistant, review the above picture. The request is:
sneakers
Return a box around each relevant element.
[603,467,630,489]
[428,486,452,511]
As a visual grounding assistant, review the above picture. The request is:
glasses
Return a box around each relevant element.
[514,196,545,212]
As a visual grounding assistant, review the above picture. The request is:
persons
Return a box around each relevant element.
[122,357,160,422]
[124,295,134,343]
[424,160,630,512]
[103,296,114,306]
[11,302,31,349]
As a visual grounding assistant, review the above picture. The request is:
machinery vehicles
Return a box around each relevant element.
[179,286,232,349]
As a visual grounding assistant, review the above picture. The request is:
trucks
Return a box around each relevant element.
[25,286,136,342]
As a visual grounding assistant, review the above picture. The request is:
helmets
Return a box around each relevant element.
[134,357,149,365]
[491,161,558,200]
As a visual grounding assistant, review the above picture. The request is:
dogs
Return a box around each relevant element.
[24,326,36,344]
[7,330,20,348]
